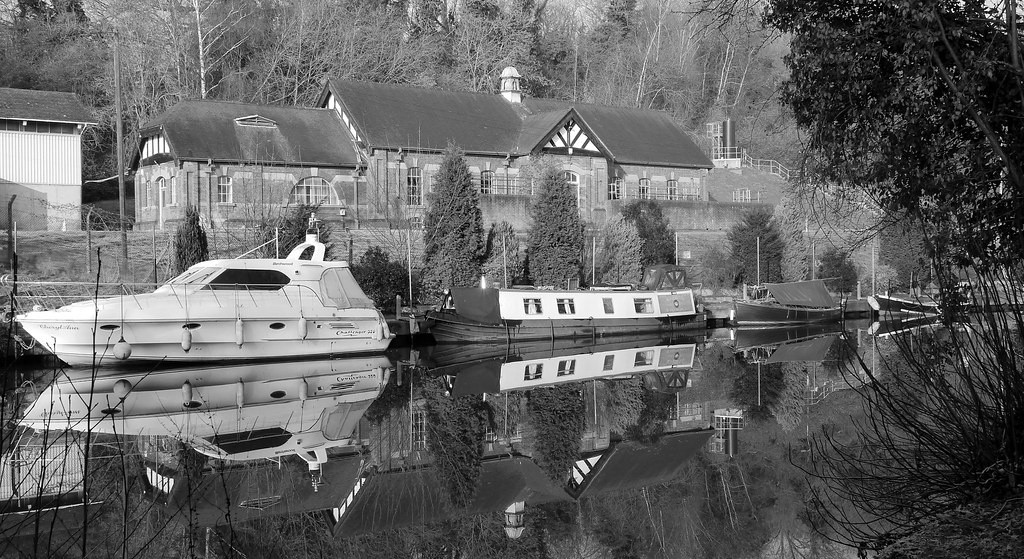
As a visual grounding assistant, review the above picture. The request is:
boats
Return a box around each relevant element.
[415,231,711,343]
[12,353,395,494]
[865,257,974,316]
[865,314,973,353]
[726,235,847,326]
[729,322,845,411]
[425,329,709,434]
[0,211,399,368]
